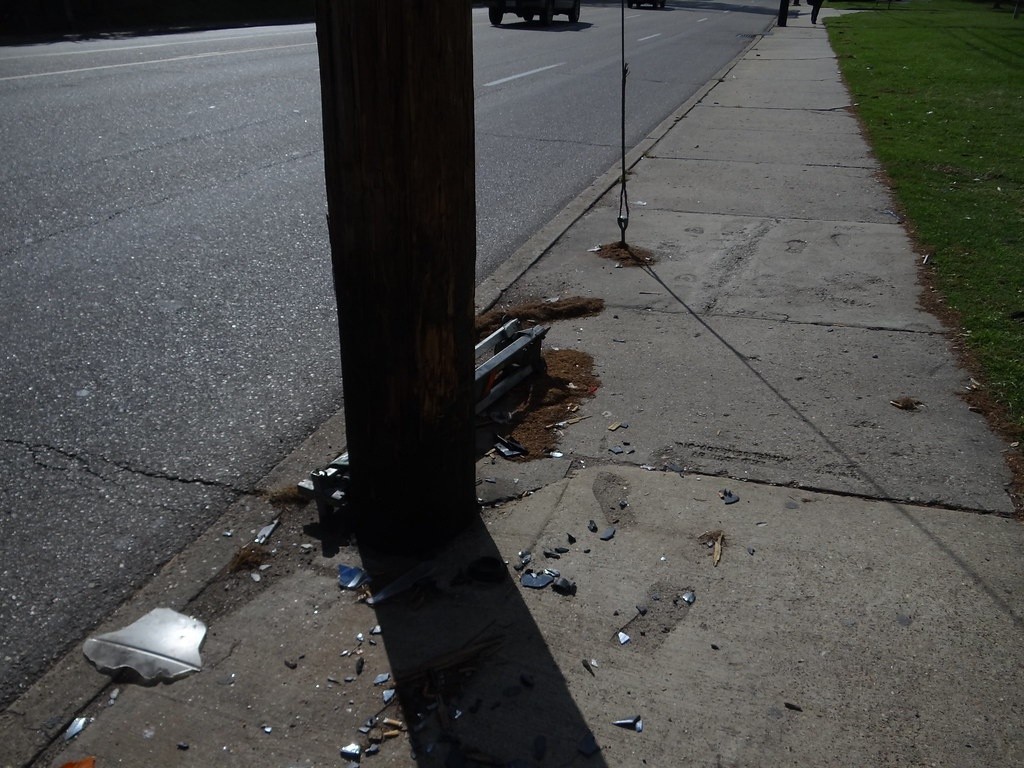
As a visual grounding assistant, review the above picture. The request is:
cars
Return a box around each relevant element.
[489,0,581,27]
